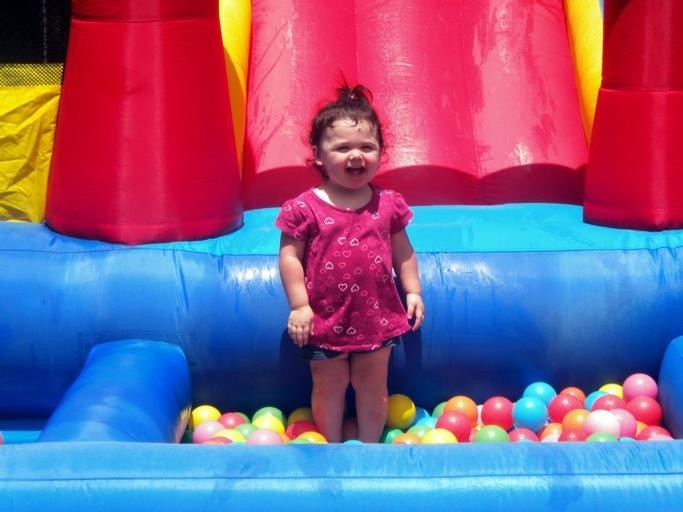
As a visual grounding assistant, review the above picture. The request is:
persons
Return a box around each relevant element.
[274,84,425,442]
